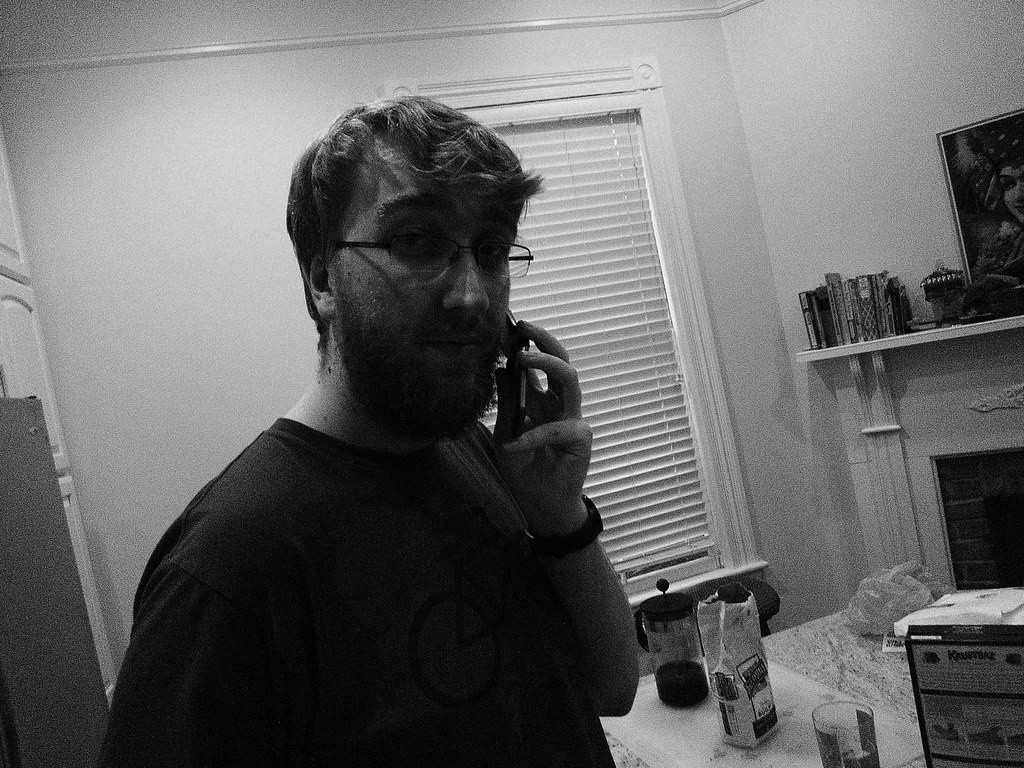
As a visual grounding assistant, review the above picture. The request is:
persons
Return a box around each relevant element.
[98,94,639,768]
[974,148,1024,281]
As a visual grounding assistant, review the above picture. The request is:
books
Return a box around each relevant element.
[799,271,902,350]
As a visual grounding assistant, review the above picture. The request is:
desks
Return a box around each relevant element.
[599,609,928,768]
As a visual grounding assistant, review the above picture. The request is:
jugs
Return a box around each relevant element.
[634,578,709,707]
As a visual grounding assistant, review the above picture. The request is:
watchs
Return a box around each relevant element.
[534,494,604,559]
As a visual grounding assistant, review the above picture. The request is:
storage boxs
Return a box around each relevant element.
[905,623,1024,768]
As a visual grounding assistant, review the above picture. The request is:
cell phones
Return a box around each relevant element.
[498,308,530,442]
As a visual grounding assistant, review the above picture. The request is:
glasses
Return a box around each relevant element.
[332,234,536,278]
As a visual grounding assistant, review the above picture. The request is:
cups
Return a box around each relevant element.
[812,701,880,768]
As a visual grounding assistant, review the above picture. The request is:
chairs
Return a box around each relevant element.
[634,576,780,652]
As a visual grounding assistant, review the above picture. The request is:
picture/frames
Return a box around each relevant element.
[935,108,1024,289]
